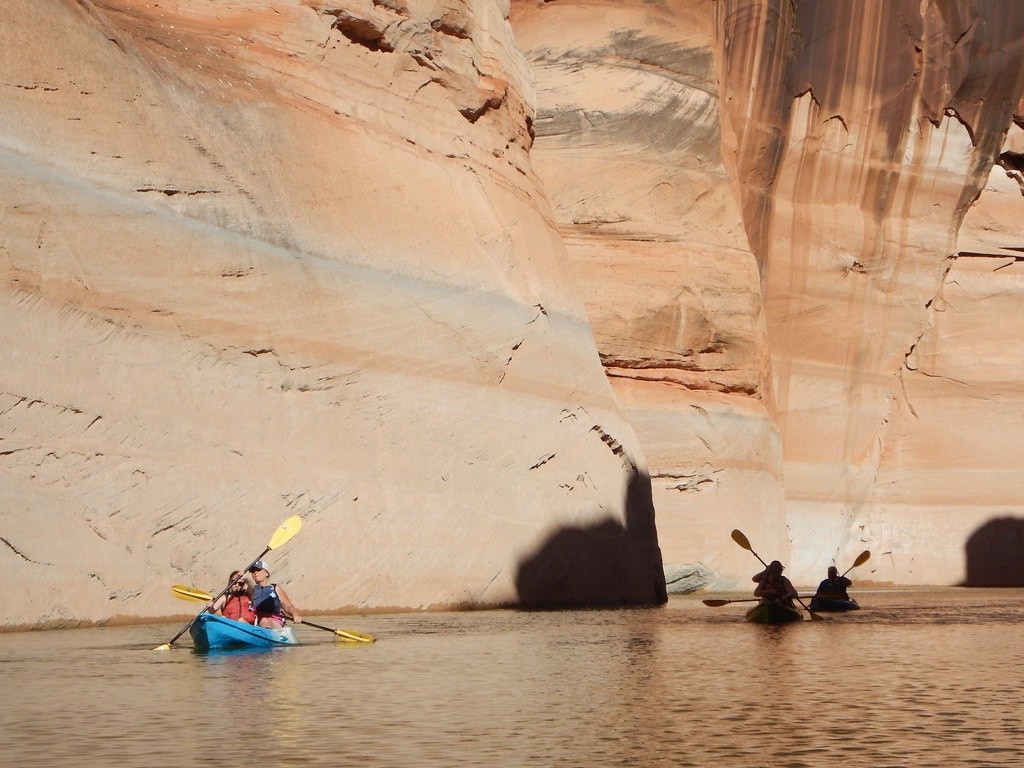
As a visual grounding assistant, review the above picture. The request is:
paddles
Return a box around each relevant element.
[731,528,825,621]
[151,514,304,653]
[703,595,814,607]
[170,582,375,645]
[806,550,871,610]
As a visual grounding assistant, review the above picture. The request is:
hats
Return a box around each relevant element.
[249,560,270,578]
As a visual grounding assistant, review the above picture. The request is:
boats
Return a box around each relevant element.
[808,596,861,615]
[188,613,302,650]
[744,595,803,625]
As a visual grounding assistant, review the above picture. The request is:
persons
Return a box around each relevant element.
[248,561,301,629]
[817,566,852,600]
[206,570,256,625]
[752,560,798,609]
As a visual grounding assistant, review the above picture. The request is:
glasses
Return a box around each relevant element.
[230,579,245,587]
